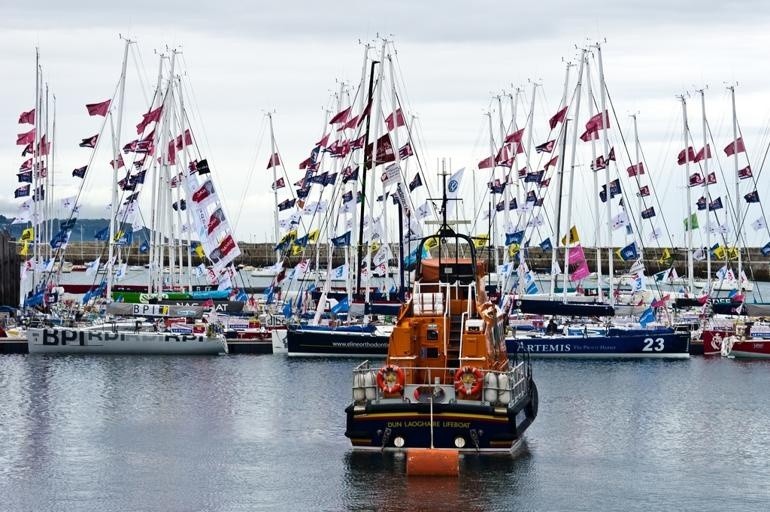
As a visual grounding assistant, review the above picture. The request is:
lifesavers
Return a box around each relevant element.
[376,365,405,395]
[454,366,484,396]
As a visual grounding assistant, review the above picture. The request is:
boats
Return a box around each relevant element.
[344,158,538,475]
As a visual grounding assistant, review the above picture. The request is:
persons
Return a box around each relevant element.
[545,319,558,335]
[158,316,171,333]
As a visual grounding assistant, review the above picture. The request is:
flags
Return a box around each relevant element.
[14,101,243,308]
[264,99,468,325]
[475,104,768,329]
